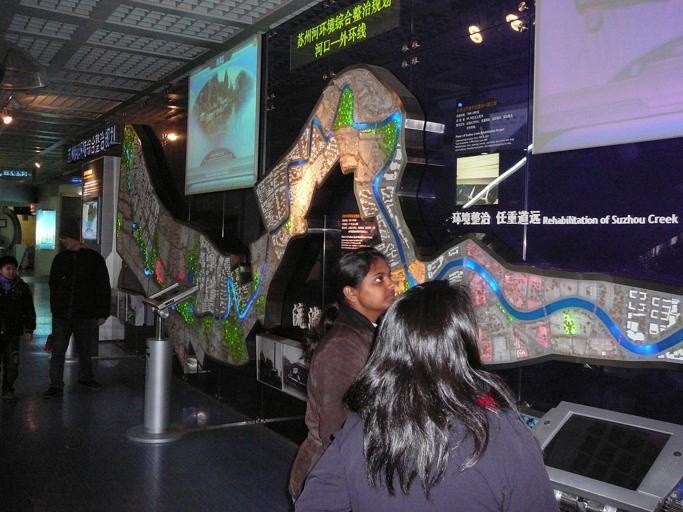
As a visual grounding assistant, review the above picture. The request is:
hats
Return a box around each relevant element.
[58,212,79,239]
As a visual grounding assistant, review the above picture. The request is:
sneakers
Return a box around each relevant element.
[1,378,105,401]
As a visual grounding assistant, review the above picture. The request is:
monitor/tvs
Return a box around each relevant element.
[143,282,200,310]
[532,401,683,512]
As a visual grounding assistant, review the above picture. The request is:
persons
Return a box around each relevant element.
[293,278,563,511]
[0,254,36,401]
[42,225,111,400]
[286,247,395,509]
